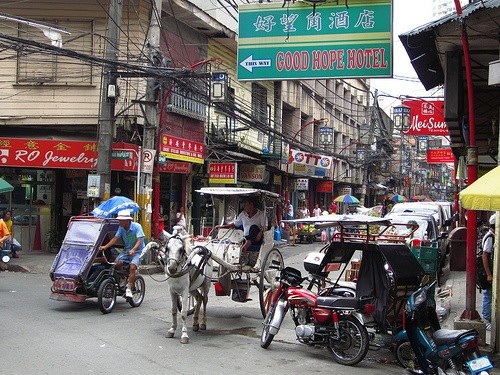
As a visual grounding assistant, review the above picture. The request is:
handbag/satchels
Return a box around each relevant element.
[476,256,493,289]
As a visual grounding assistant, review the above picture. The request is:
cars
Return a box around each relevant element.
[375,201,459,288]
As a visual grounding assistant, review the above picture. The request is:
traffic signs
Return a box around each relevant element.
[236,0,393,81]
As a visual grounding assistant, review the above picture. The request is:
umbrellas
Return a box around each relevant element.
[412,194,448,202]
[456,165,500,212]
[92,196,143,217]
[333,194,361,206]
[0,177,14,194]
[387,194,409,203]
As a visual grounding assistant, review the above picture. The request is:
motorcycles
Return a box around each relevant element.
[391,275,494,375]
[261,260,369,365]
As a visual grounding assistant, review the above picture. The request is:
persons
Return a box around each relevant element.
[475,213,497,331]
[3,209,21,257]
[0,219,10,253]
[214,196,269,253]
[176,206,186,230]
[99,210,145,298]
[278,199,339,243]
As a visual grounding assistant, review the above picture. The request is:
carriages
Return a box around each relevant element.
[140,187,285,344]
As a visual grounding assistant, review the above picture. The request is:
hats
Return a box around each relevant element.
[116,210,133,221]
[489,214,496,225]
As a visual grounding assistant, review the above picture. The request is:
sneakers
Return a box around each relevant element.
[486,322,492,330]
[126,289,132,297]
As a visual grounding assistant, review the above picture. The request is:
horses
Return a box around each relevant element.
[162,230,213,344]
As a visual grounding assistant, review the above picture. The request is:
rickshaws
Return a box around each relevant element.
[49,217,145,313]
[281,216,429,350]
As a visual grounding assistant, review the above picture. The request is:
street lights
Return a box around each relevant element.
[285,118,329,243]
[153,57,223,263]
[332,142,363,209]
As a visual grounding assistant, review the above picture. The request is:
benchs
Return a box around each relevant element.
[218,229,244,245]
